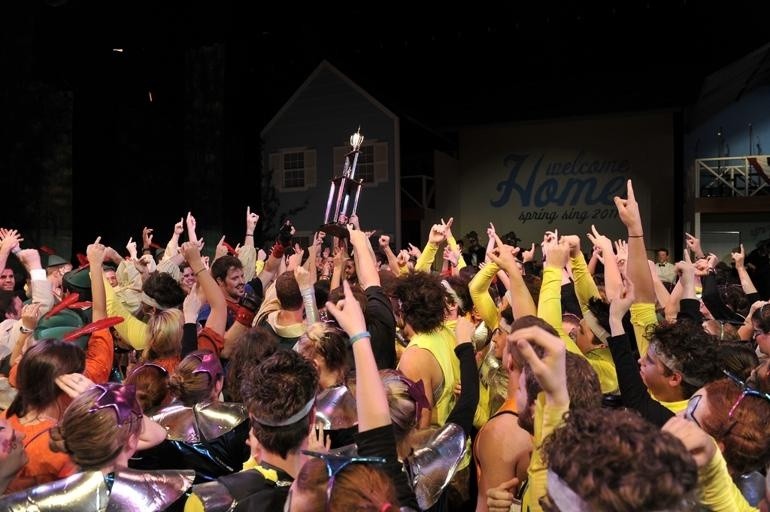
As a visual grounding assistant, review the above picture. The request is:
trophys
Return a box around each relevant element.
[320,125,365,241]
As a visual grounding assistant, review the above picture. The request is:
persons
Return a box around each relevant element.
[2,178,770,512]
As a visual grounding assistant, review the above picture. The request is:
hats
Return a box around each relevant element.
[62,267,90,292]
[41,253,69,267]
[35,308,83,339]
[38,326,89,350]
